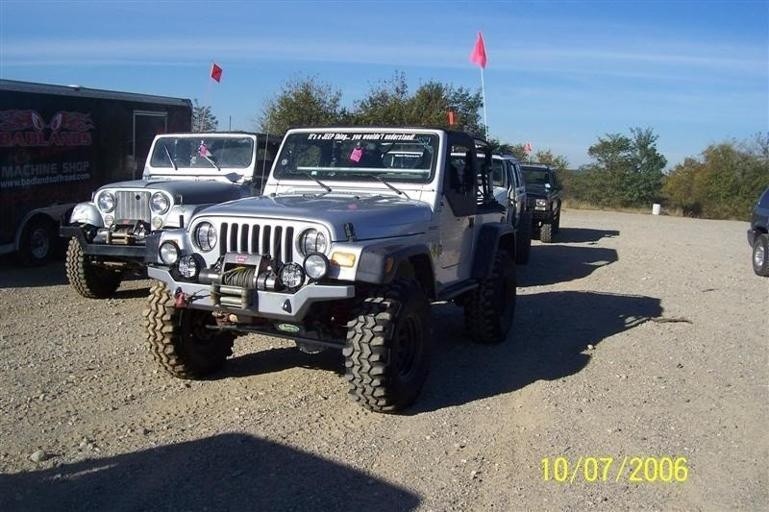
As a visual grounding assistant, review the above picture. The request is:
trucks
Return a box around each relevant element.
[0,77,194,268]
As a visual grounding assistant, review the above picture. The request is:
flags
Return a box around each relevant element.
[468,32,486,69]
[212,64,223,82]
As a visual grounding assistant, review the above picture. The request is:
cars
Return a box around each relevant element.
[745,185,769,279]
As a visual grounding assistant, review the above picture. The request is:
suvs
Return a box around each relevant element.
[516,162,562,244]
[378,150,532,266]
[147,125,522,417]
[58,129,331,301]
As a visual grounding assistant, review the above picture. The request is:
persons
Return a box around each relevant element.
[354,143,385,167]
[413,141,461,193]
